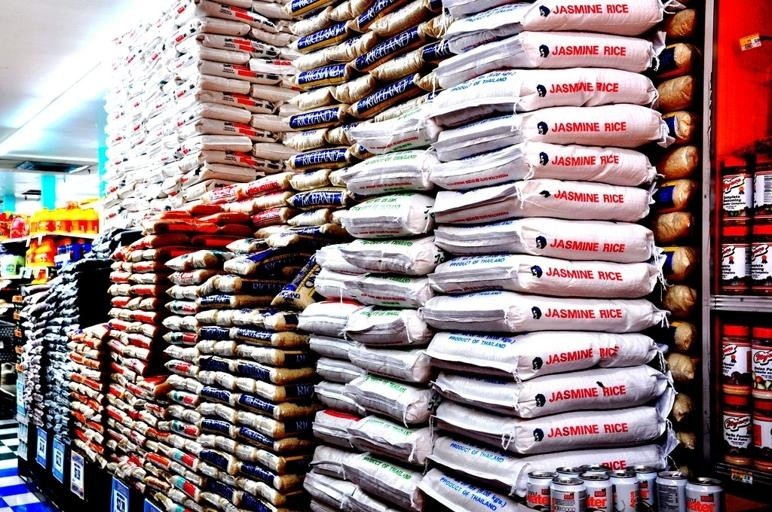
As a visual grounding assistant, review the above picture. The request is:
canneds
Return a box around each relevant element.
[723,324,753,395]
[526,467,722,512]
[719,224,751,295]
[751,327,771,399]
[722,396,753,465]
[720,166,750,219]
[752,401,772,471]
[753,458,771,498]
[753,157,772,216]
[752,225,771,296]
[722,456,749,492]
